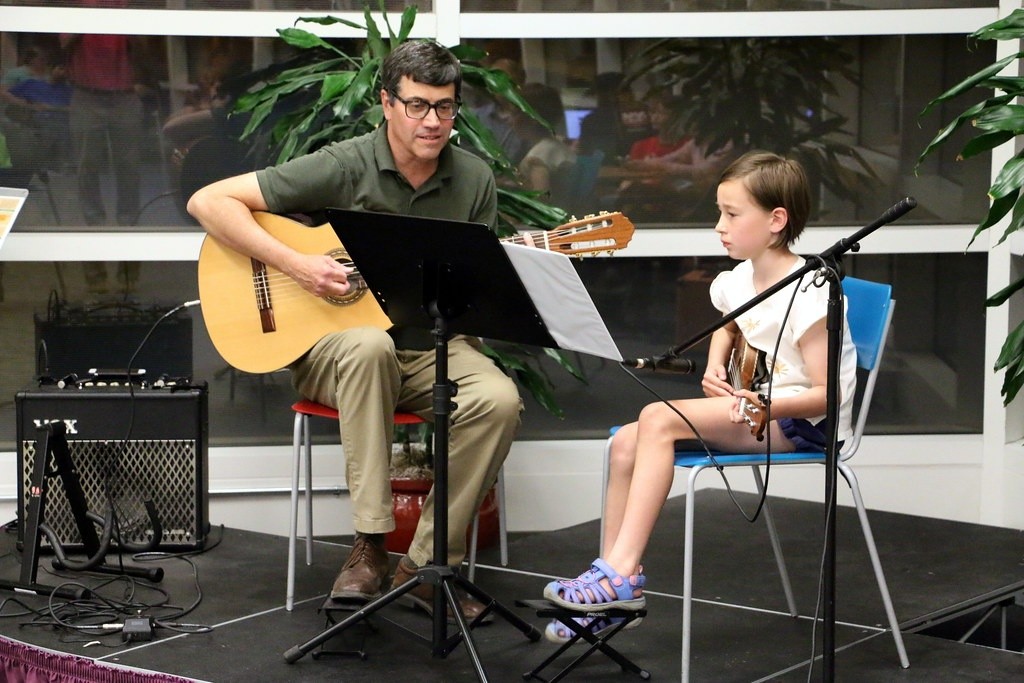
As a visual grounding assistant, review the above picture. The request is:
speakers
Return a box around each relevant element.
[14,384,211,557]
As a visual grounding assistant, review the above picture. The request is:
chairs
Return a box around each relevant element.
[610,276,910,683]
[286,403,511,608]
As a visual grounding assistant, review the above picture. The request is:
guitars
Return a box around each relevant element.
[725,326,771,443]
[195,208,634,377]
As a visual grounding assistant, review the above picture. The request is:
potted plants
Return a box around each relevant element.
[225,0,589,556]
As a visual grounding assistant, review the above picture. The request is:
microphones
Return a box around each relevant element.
[620,357,696,374]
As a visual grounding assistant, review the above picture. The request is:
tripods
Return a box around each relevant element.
[284,207,562,683]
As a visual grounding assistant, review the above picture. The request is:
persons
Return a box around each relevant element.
[455,59,593,214]
[186,40,537,627]
[543,149,857,645]
[0,0,248,304]
[578,71,736,224]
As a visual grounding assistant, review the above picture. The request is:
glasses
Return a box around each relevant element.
[382,85,463,120]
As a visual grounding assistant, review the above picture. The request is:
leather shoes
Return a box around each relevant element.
[329,532,391,601]
[389,557,494,624]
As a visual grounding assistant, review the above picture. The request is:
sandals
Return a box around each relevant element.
[544,610,643,645]
[543,557,646,612]
